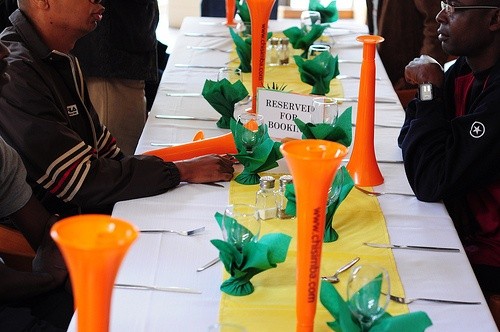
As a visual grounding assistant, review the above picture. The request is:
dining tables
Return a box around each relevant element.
[66,16,500,332]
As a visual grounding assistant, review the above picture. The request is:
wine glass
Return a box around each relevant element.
[347,264,391,332]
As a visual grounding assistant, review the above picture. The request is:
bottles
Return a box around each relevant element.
[255,174,293,220]
[266,37,290,66]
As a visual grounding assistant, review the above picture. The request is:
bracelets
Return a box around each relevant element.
[42,213,62,233]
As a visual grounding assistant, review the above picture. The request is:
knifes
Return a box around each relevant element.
[364,242,459,252]
[155,32,232,121]
[332,59,403,164]
[113,283,204,295]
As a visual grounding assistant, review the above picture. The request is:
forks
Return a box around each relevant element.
[354,185,416,198]
[138,227,205,236]
[390,295,481,305]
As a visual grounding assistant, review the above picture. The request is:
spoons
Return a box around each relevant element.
[321,257,360,283]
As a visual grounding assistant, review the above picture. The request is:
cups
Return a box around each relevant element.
[236,23,251,44]
[237,113,265,147]
[300,10,321,34]
[326,167,344,208]
[222,203,262,252]
[310,97,338,127]
[217,67,243,85]
[308,44,332,61]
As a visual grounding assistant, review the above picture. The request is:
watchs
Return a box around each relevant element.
[416,82,444,102]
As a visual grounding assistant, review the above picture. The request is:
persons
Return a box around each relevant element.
[0,0,234,207]
[0,0,235,332]
[397,0,500,296]
[365,0,449,91]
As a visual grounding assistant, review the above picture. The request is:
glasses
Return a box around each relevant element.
[441,1,498,16]
